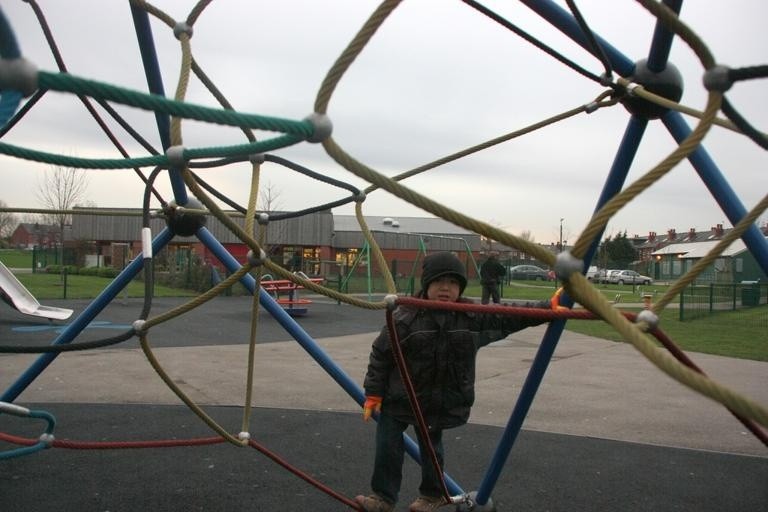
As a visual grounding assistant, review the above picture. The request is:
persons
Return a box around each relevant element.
[353,250,574,510]
[479,251,507,305]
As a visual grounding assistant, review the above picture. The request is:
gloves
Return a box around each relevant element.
[550,286,570,312]
[362,395,383,422]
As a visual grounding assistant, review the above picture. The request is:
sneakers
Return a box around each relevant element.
[354,493,394,512]
[409,493,444,512]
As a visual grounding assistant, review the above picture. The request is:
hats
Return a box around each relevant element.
[489,251,499,258]
[420,251,468,297]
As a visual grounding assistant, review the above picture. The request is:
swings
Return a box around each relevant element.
[372,231,412,292]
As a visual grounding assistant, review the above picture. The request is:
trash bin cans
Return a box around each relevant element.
[741,280,760,307]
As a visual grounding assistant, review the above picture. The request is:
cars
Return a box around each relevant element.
[510,265,654,285]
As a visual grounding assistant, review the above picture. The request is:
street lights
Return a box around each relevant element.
[559,217,565,252]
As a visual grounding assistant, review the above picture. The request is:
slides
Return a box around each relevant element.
[0,261,73,319]
